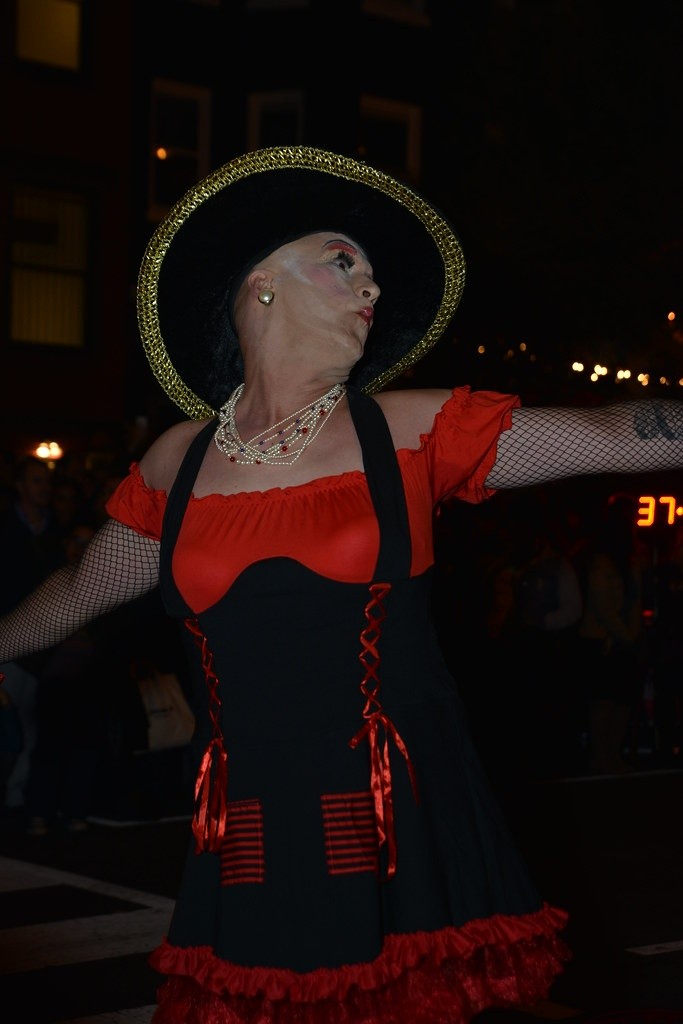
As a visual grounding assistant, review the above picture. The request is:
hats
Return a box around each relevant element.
[137,148,466,419]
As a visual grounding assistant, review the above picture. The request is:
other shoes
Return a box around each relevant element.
[61,818,86,831]
[24,817,48,834]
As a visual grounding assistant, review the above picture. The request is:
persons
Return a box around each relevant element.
[0,442,683,1023]
[0,146,683,1023]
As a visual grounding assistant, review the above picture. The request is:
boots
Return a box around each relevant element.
[590,700,630,774]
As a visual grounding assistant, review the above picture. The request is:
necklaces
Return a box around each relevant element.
[216,383,347,467]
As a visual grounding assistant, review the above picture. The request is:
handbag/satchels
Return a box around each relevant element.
[130,657,195,755]
